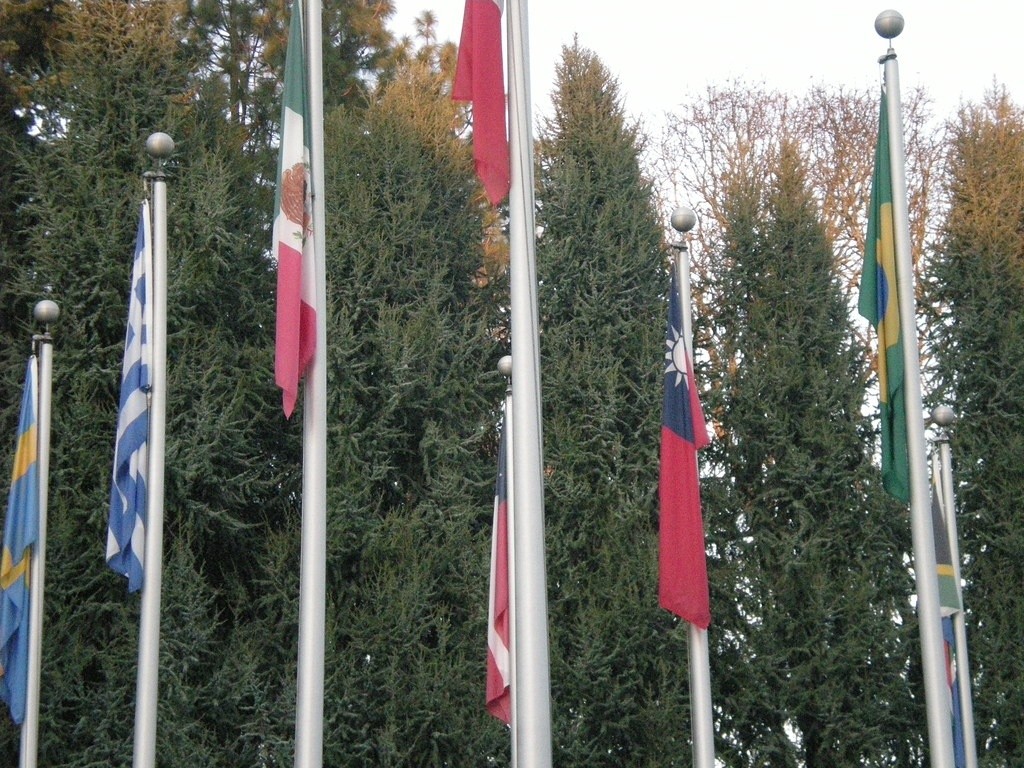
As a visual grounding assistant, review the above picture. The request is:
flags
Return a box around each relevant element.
[484,409,511,723]
[657,264,711,629]
[857,86,910,503]
[451,0,510,205]
[932,452,965,768]
[106,199,154,592]
[0,355,38,724]
[272,0,317,420]
